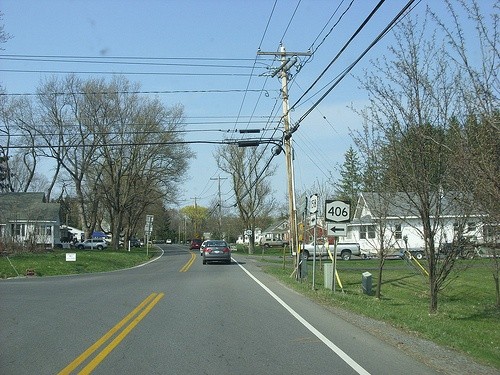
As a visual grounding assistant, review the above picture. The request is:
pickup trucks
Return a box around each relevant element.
[291,237,361,261]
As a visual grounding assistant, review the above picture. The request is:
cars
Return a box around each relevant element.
[187,238,212,256]
[201,239,232,264]
[104,234,165,248]
[165,238,172,244]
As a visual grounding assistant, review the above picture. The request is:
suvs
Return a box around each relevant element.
[74,238,109,251]
[260,237,289,248]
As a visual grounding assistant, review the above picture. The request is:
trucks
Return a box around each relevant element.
[424,219,500,254]
